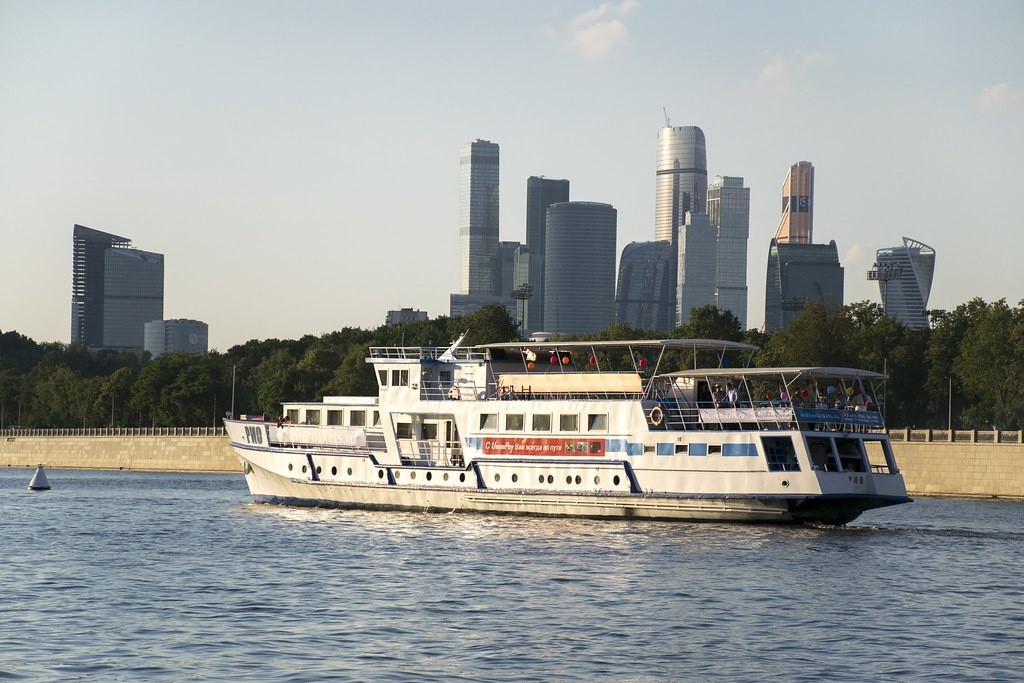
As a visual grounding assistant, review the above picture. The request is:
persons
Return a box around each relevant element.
[712,379,876,472]
[277,416,286,429]
[283,416,294,428]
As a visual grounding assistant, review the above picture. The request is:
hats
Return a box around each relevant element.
[714,384,721,389]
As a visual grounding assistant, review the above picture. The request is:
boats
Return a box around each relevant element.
[224,331,912,530]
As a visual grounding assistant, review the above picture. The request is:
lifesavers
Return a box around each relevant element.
[650,406,665,426]
[448,385,460,400]
